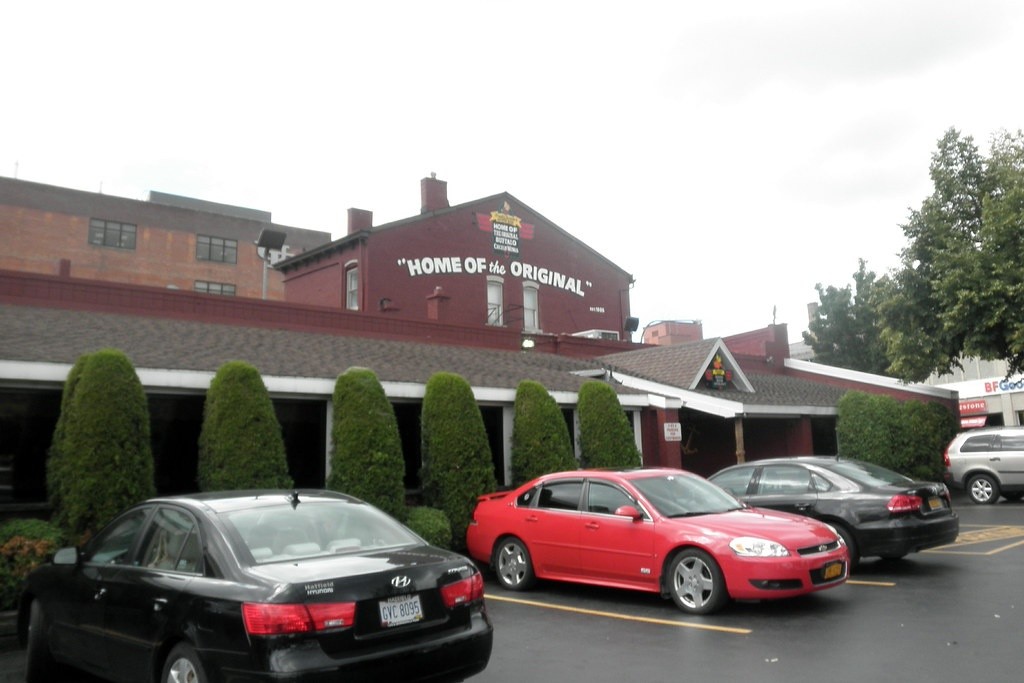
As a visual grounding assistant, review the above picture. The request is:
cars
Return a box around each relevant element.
[463,464,851,617]
[708,455,958,573]
[17,487,495,683]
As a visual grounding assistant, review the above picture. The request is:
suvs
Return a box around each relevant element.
[943,426,1024,505]
